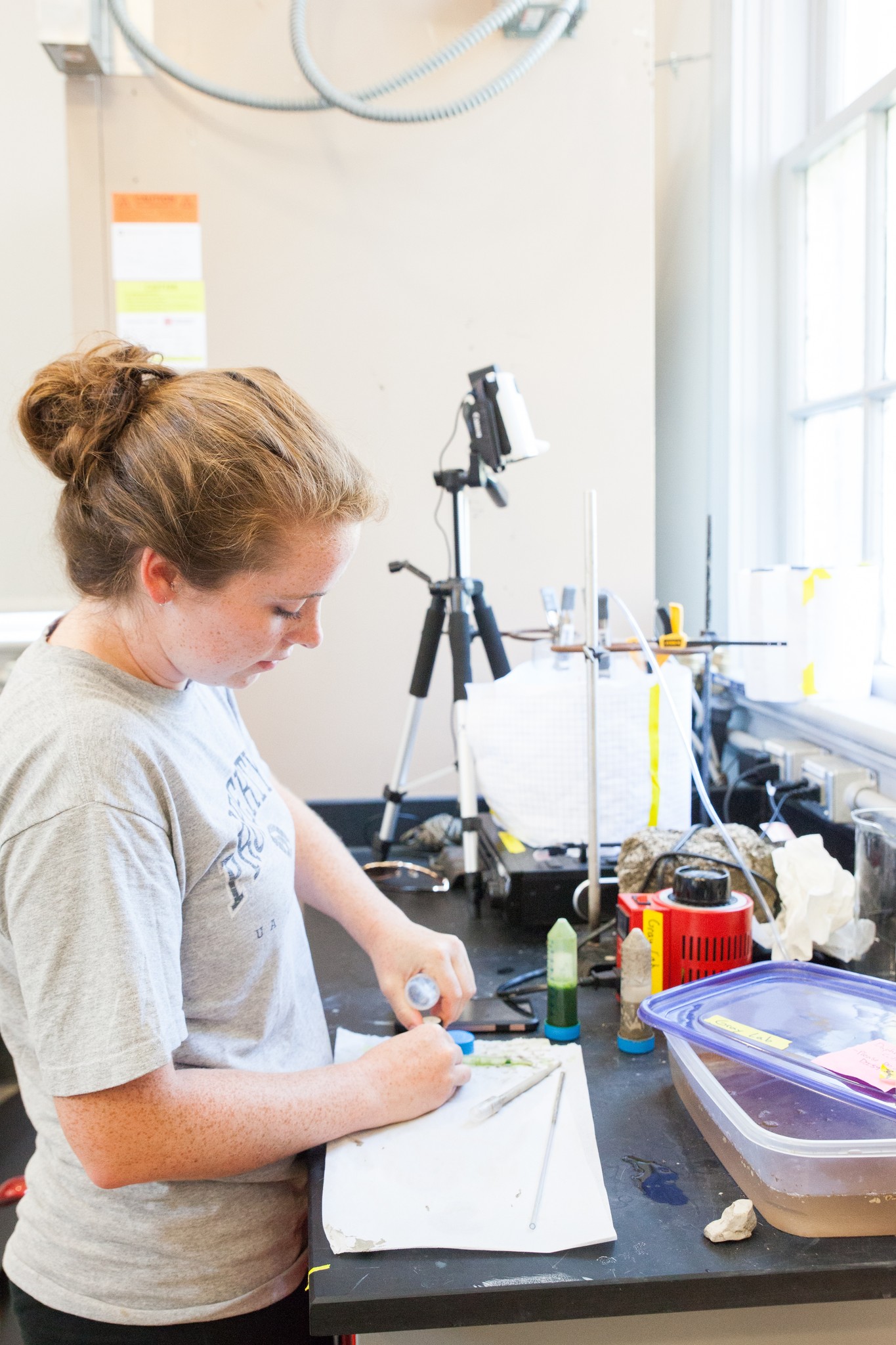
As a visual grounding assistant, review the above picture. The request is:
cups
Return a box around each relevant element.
[852,808,896,986]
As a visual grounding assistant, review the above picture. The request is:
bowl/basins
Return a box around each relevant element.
[668,1026,895,1236]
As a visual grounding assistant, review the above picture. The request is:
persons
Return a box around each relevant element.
[2,337,481,1345]
